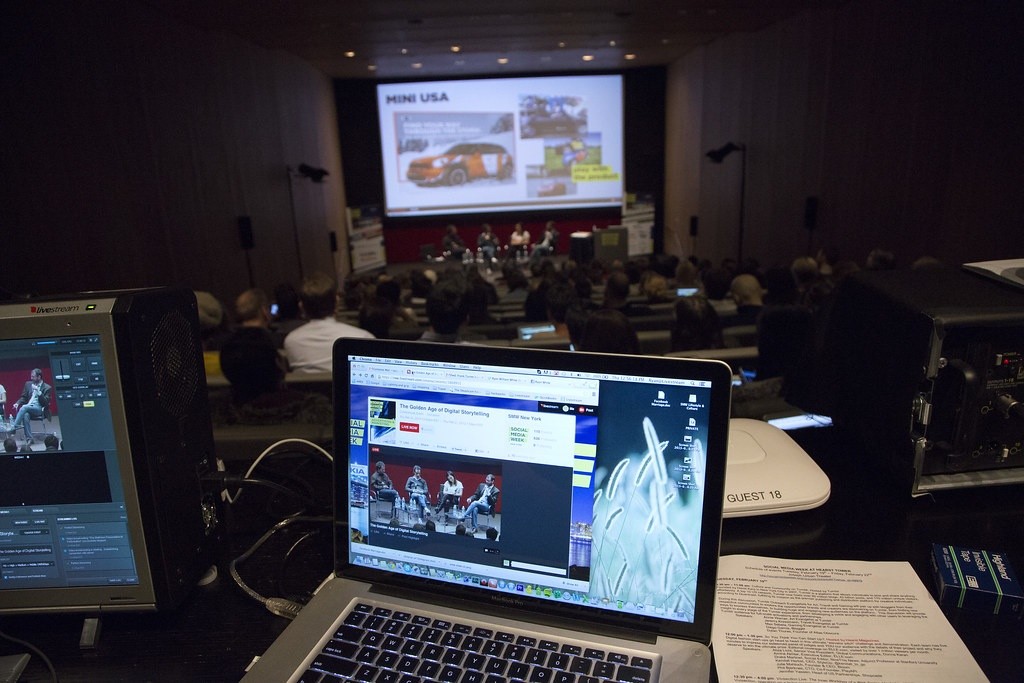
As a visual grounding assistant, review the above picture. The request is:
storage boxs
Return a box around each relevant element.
[930,541,1023,622]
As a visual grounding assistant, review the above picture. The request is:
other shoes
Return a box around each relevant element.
[457,517,465,522]
[426,511,430,517]
[25,439,33,445]
[419,518,422,524]
[471,528,477,534]
[436,507,439,514]
[10,428,16,435]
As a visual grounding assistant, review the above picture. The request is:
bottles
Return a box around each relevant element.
[395,497,416,510]
[9,414,13,426]
[462,506,465,517]
[453,504,456,516]
[0,415,3,427]
[443,245,528,262]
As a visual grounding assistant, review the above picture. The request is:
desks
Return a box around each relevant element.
[0,425,24,451]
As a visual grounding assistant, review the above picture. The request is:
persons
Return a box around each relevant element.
[390,518,399,525]
[419,259,641,356]
[193,266,432,427]
[458,474,499,533]
[442,221,558,272]
[0,367,64,452]
[435,470,464,525]
[413,523,426,532]
[465,532,474,538]
[455,524,466,536]
[425,520,435,532]
[404,465,431,523]
[622,252,946,377]
[485,527,498,541]
[370,460,409,519]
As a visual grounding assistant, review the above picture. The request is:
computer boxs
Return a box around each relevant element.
[0,284,228,621]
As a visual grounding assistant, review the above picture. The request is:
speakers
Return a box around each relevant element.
[801,197,819,231]
[239,216,255,250]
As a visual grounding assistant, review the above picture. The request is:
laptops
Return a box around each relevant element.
[239,336,735,683]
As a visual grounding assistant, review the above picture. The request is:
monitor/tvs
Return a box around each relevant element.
[0,298,175,649]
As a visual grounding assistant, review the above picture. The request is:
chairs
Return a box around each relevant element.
[204,294,754,419]
[369,483,497,529]
[14,402,57,439]
